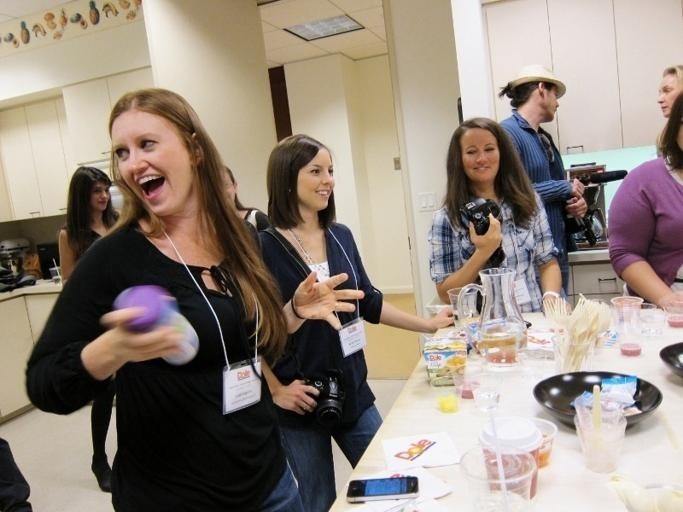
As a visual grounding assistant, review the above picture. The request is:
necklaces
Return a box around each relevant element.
[289,228,330,275]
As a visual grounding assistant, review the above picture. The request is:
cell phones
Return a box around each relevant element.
[346,476,419,503]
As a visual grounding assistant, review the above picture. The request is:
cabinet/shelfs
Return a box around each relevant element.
[565,262,631,308]
[487,0,682,154]
[1,71,68,222]
[61,68,152,172]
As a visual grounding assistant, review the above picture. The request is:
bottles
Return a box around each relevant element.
[113,285,200,366]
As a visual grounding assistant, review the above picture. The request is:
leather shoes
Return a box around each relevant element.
[92,455,113,491]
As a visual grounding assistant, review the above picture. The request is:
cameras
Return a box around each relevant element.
[311,368,346,427]
[460,197,504,235]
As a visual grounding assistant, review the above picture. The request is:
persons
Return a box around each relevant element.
[606,92,683,316]
[0,439,35,512]
[262,134,455,511]
[653,64,682,158]
[427,117,563,312]
[55,165,121,493]
[499,65,588,302]
[223,164,271,233]
[23,89,365,512]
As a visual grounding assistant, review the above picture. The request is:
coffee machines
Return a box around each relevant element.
[565,165,609,244]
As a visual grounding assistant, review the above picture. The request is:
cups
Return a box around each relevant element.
[49,267,61,285]
[430,267,683,512]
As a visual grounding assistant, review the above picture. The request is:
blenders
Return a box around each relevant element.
[0,238,32,285]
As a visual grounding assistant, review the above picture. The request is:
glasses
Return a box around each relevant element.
[541,134,555,162]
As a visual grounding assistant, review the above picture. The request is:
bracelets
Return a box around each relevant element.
[291,298,305,321]
[542,291,560,297]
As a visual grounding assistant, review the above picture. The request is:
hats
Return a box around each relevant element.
[502,64,567,97]
